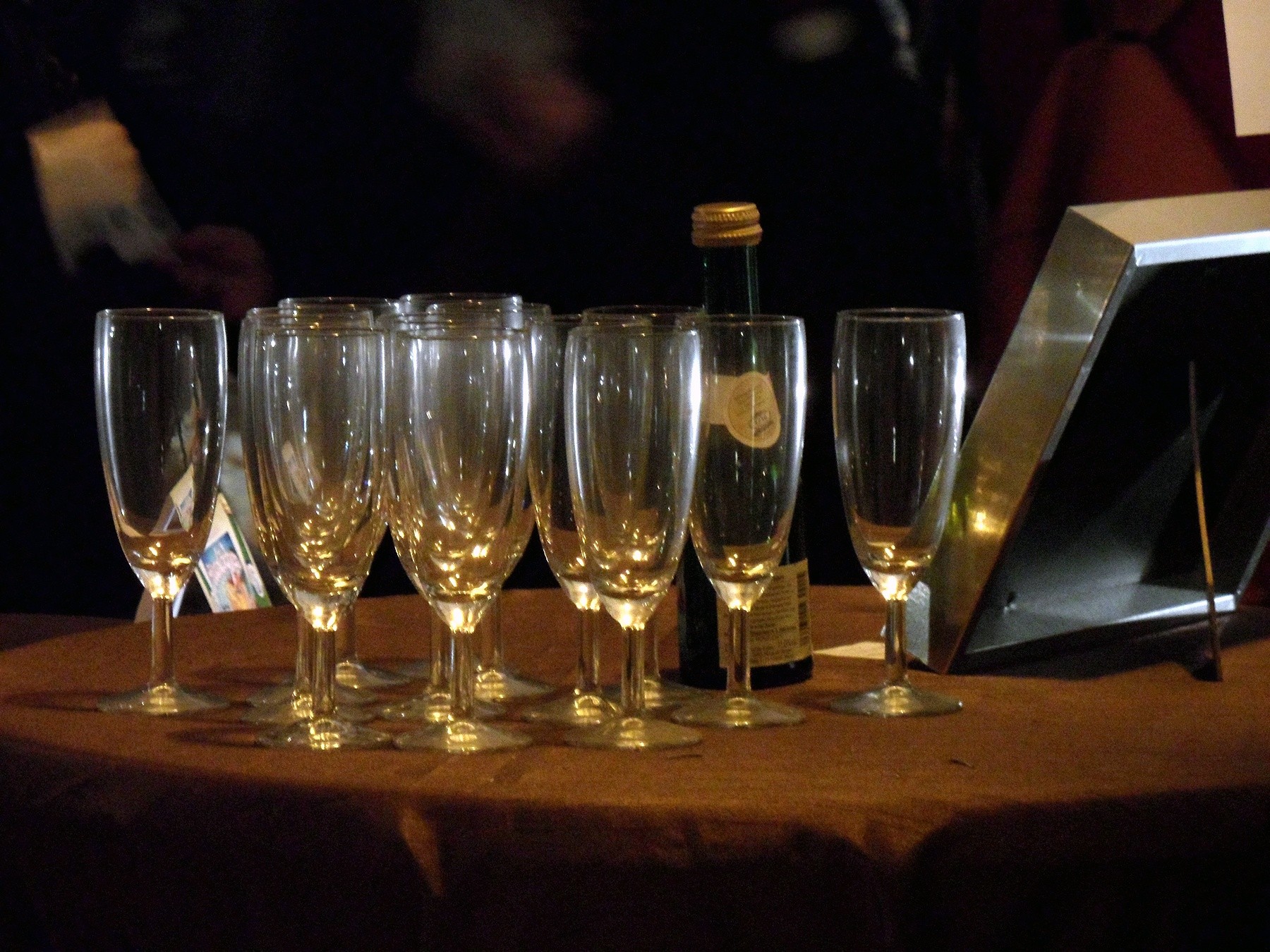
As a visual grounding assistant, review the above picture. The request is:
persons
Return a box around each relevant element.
[154,0,973,581]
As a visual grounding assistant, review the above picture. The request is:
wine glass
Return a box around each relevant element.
[666,312,807,728]
[93,307,231,717]
[833,306,968,719]
[562,322,703,753]
[235,292,561,753]
[530,303,709,723]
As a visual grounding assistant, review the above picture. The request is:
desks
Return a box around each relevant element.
[0,585,1270,952]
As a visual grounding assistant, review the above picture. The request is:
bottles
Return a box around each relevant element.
[675,201,815,690]
